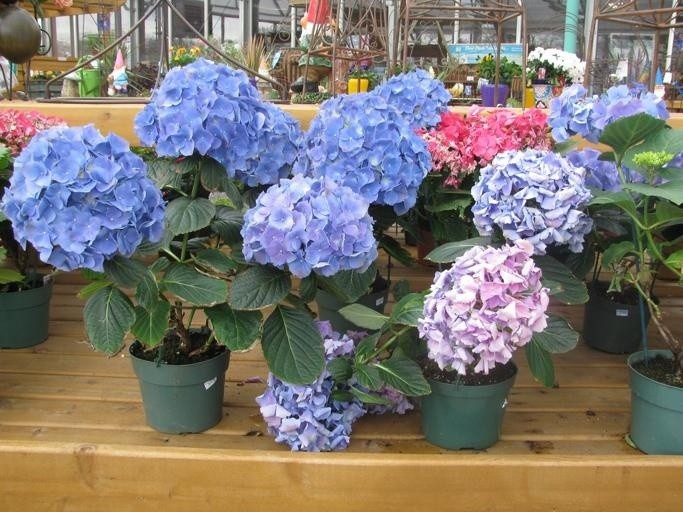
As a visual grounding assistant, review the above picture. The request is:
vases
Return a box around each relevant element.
[414,361,516,451]
[128,328,231,434]
[1,273,55,351]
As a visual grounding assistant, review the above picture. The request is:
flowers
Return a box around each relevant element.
[545,82,668,146]
[241,176,378,279]
[299,93,431,213]
[135,59,302,184]
[470,150,592,252]
[254,321,412,453]
[416,107,550,186]
[366,67,449,129]
[1,107,166,275]
[528,49,587,84]
[562,144,682,190]
[415,241,550,374]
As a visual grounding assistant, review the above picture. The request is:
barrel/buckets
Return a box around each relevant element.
[60,78,78,97]
[78,68,101,97]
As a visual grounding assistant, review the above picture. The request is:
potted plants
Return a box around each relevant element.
[582,112,683,458]
[475,54,521,107]
